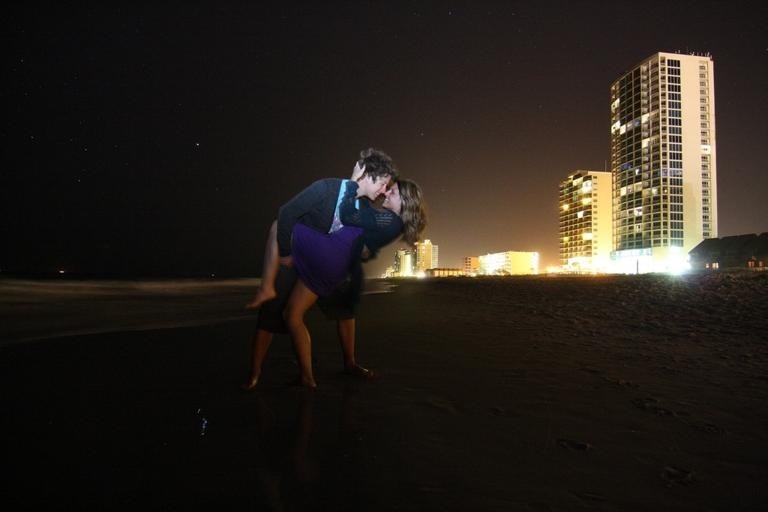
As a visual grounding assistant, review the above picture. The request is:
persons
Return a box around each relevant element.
[242,148,393,391]
[245,179,427,388]
